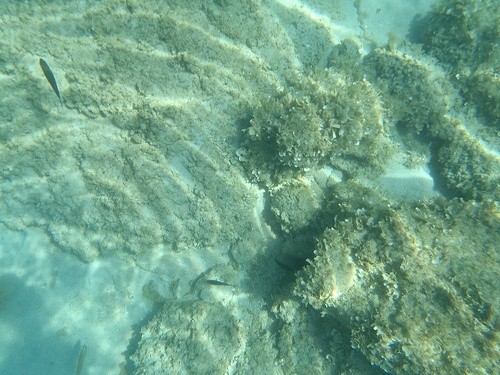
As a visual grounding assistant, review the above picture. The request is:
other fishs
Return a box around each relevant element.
[39,58,60,100]
[274,259,294,272]
[206,280,237,288]
